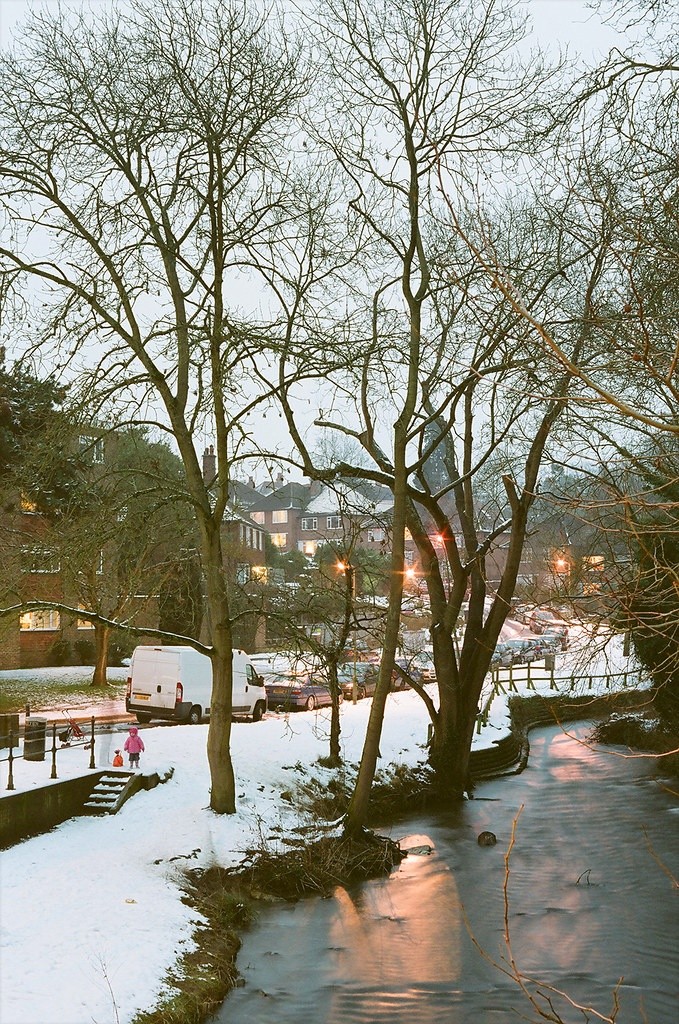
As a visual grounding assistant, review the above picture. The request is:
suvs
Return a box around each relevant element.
[327,640,380,662]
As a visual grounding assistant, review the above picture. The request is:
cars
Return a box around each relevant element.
[526,638,555,661]
[514,604,541,625]
[370,658,426,690]
[529,610,555,634]
[542,628,568,651]
[410,647,462,683]
[265,671,344,711]
[339,662,395,700]
[489,642,515,671]
[418,584,427,593]
[539,636,562,654]
[507,639,539,664]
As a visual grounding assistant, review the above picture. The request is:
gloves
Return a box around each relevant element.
[142,747,145,752]
[124,748,128,751]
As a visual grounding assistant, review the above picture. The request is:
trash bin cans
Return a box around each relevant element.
[23,716,47,761]
[545,653,555,671]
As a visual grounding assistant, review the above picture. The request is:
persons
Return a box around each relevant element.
[124,727,145,768]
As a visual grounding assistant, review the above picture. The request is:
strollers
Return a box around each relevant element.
[60,707,94,750]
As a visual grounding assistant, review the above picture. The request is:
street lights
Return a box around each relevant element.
[558,560,570,596]
[542,585,552,609]
[339,563,358,707]
[253,566,268,585]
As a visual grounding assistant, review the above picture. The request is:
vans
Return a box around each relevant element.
[509,597,523,616]
[125,643,269,723]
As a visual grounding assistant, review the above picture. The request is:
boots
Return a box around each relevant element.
[129,761,134,769]
[135,761,140,769]
